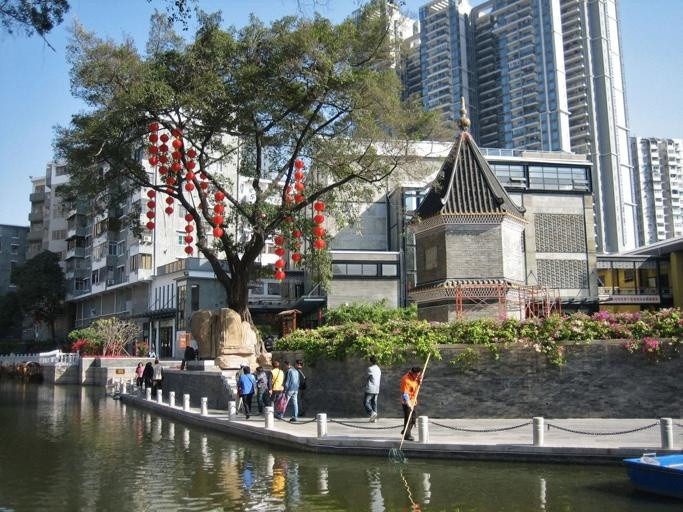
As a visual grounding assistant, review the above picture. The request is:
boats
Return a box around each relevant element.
[16,361,42,383]
[622,452,683,498]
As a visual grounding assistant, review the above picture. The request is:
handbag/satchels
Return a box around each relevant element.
[256,378,265,388]
[262,391,271,406]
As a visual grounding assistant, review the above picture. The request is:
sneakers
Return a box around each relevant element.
[401,431,414,440]
[246,415,250,419]
[290,418,297,422]
[368,412,377,422]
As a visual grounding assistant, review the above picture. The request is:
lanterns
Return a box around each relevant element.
[274,236,283,246]
[275,248,285,259]
[275,260,286,270]
[313,215,324,226]
[312,225,325,239]
[146,123,225,260]
[275,271,285,283]
[284,159,304,261]
[313,239,325,255]
[314,200,325,214]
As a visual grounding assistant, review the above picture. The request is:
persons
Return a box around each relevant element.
[148,348,155,359]
[136,362,144,388]
[237,367,256,420]
[402,464,431,512]
[142,409,153,444]
[256,366,267,414]
[179,346,194,369]
[220,439,299,512]
[364,461,386,512]
[131,408,144,446]
[294,359,306,418]
[151,415,162,447]
[142,362,153,391]
[357,355,381,425]
[234,363,246,416]
[273,360,299,422]
[152,359,163,397]
[184,346,190,361]
[255,369,273,412]
[271,360,284,415]
[399,365,421,442]
